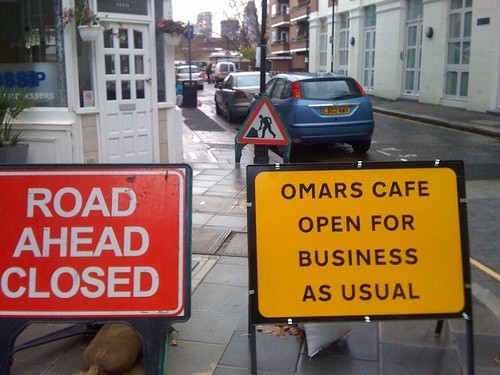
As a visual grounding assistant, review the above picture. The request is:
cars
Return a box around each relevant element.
[176,65,205,90]
[214,70,275,122]
[247,73,375,155]
[174,59,207,73]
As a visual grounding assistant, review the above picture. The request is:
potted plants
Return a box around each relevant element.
[0,89,31,164]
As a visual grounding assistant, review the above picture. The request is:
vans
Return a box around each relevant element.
[213,61,237,87]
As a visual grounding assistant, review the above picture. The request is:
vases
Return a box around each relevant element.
[157,18,188,46]
[63,0,124,41]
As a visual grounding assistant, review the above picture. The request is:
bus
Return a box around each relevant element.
[208,50,243,72]
